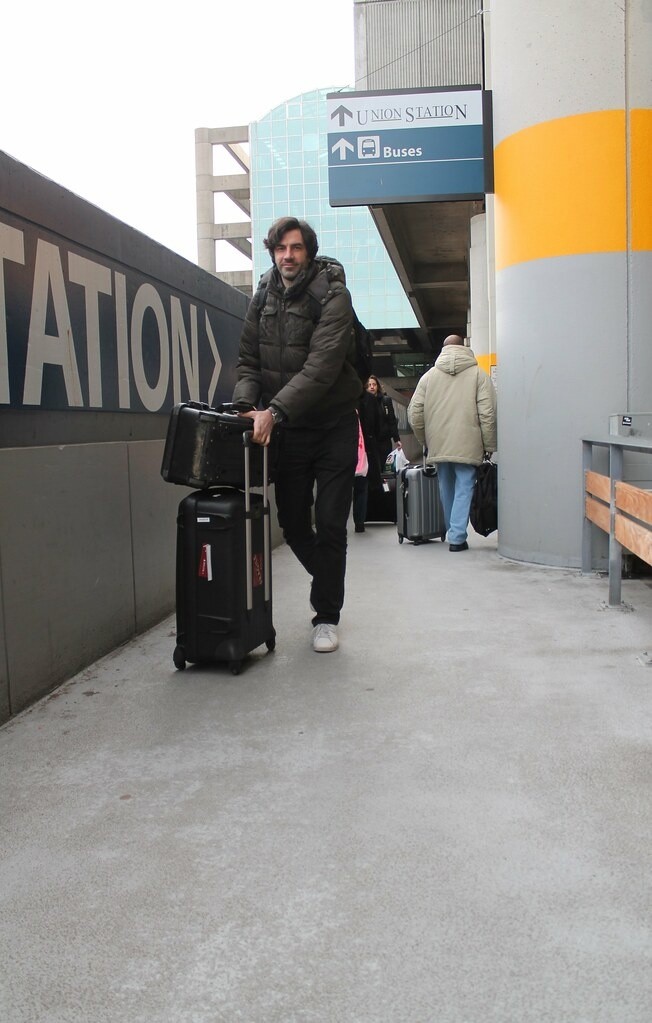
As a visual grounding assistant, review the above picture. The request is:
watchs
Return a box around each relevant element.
[268,407,283,424]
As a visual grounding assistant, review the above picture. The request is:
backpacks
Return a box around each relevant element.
[255,279,370,386]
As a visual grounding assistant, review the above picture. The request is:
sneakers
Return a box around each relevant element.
[310,624,339,652]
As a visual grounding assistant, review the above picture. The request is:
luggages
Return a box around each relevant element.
[396,445,446,545]
[173,430,276,675]
[364,465,397,523]
[159,400,282,490]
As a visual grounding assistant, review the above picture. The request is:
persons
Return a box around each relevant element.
[353,374,402,532]
[231,217,359,652]
[407,335,497,551]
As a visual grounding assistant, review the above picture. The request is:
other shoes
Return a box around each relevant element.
[449,540,468,552]
[354,520,365,533]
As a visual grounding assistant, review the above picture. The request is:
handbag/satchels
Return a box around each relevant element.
[384,446,410,473]
[469,452,498,538]
